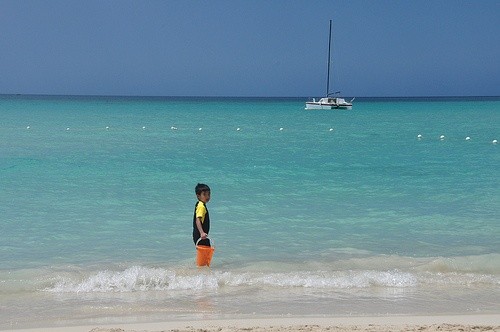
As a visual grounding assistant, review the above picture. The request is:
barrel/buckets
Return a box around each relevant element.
[195,236,215,268]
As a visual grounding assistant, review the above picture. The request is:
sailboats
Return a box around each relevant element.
[305,20,352,109]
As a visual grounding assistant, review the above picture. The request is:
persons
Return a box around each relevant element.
[193,183,211,249]
